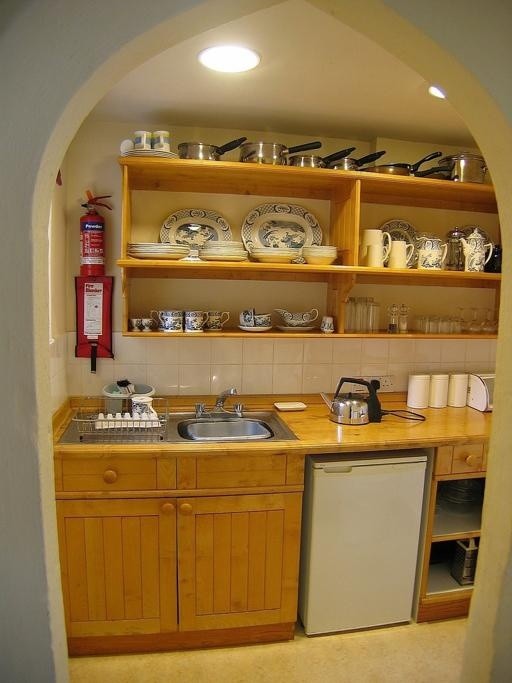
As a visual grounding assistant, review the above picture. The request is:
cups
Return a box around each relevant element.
[273,308,318,327]
[150,310,229,332]
[360,229,391,267]
[343,297,380,333]
[133,130,170,152]
[239,307,272,326]
[386,241,414,268]
[417,316,461,334]
[131,397,158,417]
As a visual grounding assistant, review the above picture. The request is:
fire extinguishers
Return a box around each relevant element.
[79,191,112,277]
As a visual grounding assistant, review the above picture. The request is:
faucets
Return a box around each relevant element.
[216,388,243,418]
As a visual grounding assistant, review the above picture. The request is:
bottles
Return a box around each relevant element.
[448,226,465,270]
[388,304,409,333]
[406,373,470,407]
[320,316,335,332]
[95,413,162,429]
[485,244,501,273]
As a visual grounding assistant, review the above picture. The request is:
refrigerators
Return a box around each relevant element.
[299,449,430,637]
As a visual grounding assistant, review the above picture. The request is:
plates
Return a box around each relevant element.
[240,202,322,265]
[439,483,474,504]
[120,149,180,159]
[273,401,308,411]
[126,242,191,260]
[379,219,421,269]
[276,325,316,331]
[251,247,299,264]
[197,240,249,261]
[302,246,338,265]
[236,326,272,332]
[159,208,233,261]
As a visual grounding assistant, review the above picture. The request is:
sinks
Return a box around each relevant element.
[167,410,300,441]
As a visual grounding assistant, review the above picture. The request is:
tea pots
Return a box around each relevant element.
[319,377,382,425]
[460,227,493,272]
[415,236,448,270]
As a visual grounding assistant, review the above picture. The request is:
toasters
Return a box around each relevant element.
[468,370,495,413]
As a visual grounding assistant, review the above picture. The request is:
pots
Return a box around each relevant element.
[240,141,322,166]
[289,146,356,169]
[367,151,444,176]
[326,150,386,170]
[179,136,248,161]
[438,151,487,184]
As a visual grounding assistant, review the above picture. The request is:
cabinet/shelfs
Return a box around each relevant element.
[116,154,502,338]
[55,445,304,638]
[412,443,489,624]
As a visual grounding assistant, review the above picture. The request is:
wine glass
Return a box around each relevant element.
[458,308,498,335]
[128,318,153,332]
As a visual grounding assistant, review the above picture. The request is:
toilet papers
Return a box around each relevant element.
[407,374,430,409]
[430,373,449,408]
[449,373,470,407]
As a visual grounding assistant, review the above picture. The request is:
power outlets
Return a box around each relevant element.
[350,375,396,393]
